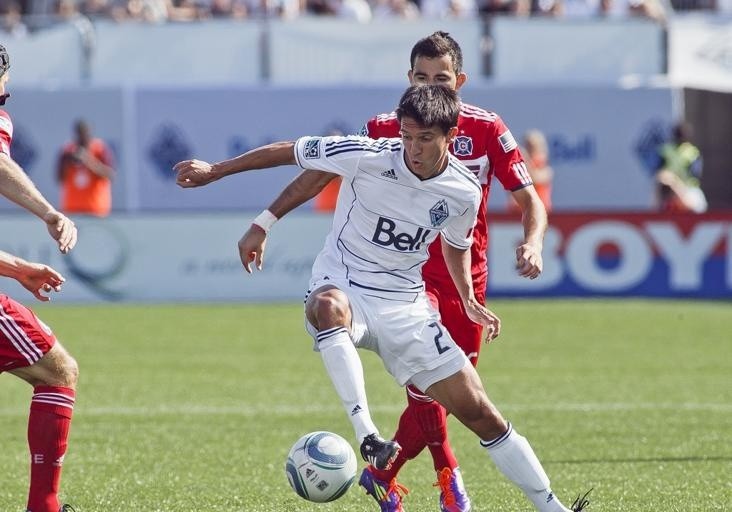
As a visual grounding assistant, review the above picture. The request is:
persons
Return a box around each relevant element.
[0,44,89,511]
[54,117,120,219]
[313,127,350,210]
[236,31,548,512]
[505,126,555,212]
[654,122,703,213]
[170,82,583,512]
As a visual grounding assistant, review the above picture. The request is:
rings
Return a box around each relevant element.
[487,323,493,329]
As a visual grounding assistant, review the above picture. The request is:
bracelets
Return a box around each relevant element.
[254,205,280,235]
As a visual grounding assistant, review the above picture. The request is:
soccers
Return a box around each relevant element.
[285,432,357,503]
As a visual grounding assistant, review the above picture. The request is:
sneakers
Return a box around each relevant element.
[359,468,408,512]
[360,433,402,471]
[433,467,471,512]
